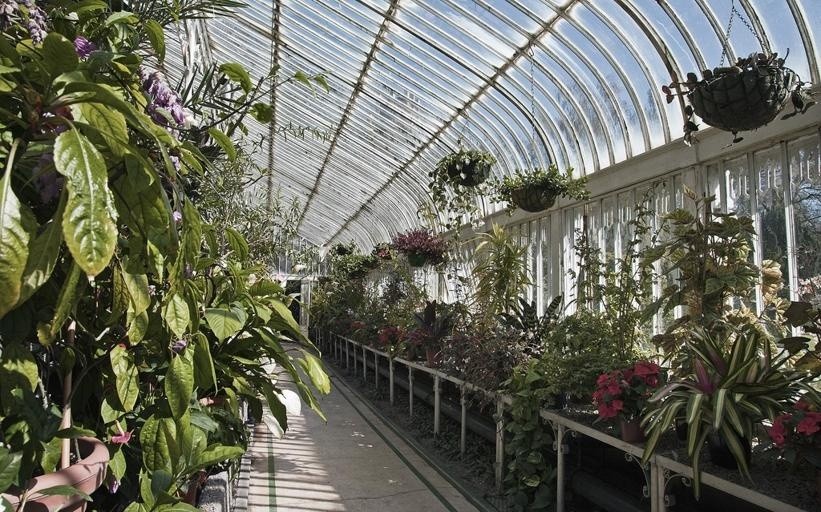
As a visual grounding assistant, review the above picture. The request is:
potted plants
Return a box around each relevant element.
[415,138,496,233]
[390,178,819,510]
[659,54,819,147]
[491,165,594,219]
